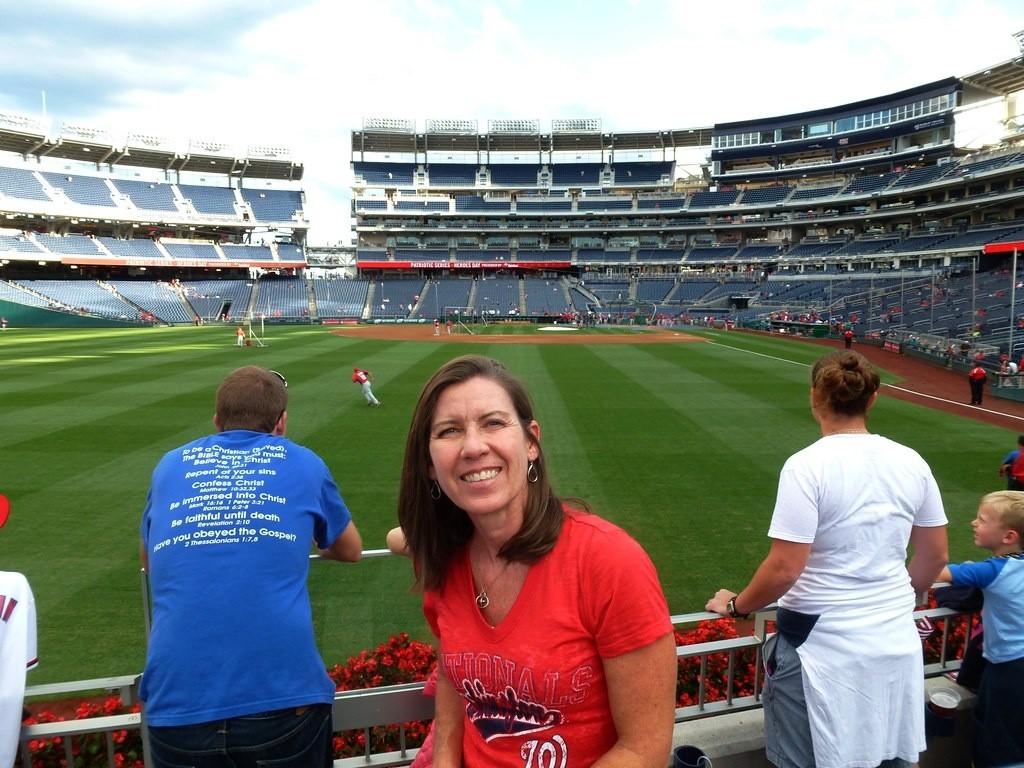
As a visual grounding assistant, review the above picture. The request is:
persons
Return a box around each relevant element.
[807,210,812,216]
[137,364,362,768]
[385,355,679,768]
[192,293,205,298]
[0,487,43,768]
[496,255,504,261]
[133,306,154,320]
[235,327,245,346]
[936,489,1024,768]
[1,315,8,328]
[749,265,755,271]
[170,278,181,288]
[258,311,263,317]
[998,435,1024,491]
[48,300,90,313]
[706,348,950,768]
[222,314,229,321]
[275,281,1024,406]
[195,316,199,326]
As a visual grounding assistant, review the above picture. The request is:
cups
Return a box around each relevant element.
[928,686,961,717]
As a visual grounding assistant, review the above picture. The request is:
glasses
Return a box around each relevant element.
[270,370,288,424]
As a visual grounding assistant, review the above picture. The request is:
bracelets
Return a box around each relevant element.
[997,470,1003,472]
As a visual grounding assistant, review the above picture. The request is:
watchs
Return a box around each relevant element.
[727,595,750,618]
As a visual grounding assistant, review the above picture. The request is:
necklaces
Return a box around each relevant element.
[475,541,512,609]
